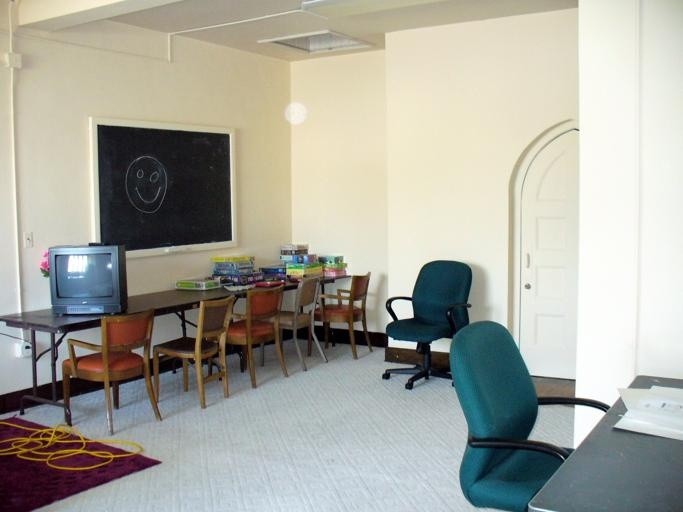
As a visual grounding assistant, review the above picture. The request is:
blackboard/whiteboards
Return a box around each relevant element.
[88,116,238,260]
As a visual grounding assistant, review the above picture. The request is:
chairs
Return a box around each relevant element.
[208,284,288,388]
[154,295,235,408]
[449,321,612,512]
[260,278,327,371]
[382,261,471,389]
[307,272,373,360]
[61,308,161,436]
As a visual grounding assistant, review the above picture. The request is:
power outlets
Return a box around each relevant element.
[16,342,33,359]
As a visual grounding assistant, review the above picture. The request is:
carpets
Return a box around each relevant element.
[0,417,162,512]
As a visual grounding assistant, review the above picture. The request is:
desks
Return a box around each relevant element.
[0,273,351,424]
[528,375,682,512]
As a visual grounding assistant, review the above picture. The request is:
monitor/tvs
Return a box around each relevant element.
[49,245,128,318]
